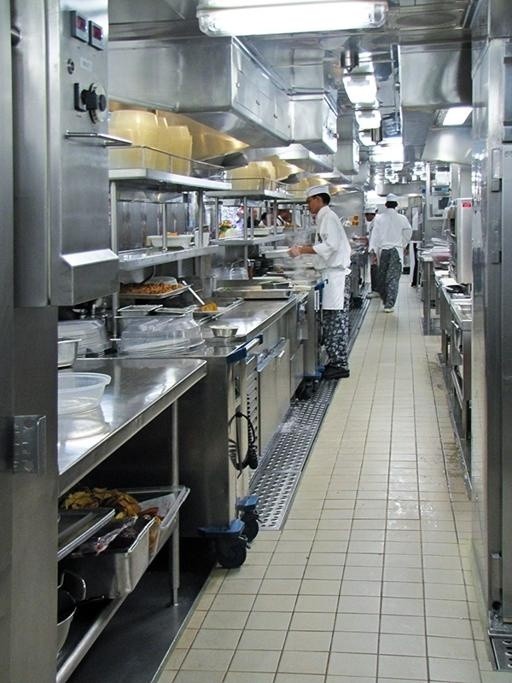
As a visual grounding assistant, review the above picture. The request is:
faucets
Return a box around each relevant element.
[231,259,251,267]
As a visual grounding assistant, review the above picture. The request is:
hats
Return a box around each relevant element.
[363,205,376,214]
[307,185,330,198]
[386,193,397,201]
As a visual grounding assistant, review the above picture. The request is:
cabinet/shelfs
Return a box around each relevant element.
[107,36,342,155]
[105,167,313,345]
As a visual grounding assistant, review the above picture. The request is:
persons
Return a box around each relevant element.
[367,193,414,314]
[257,212,275,227]
[287,185,354,383]
[274,209,291,226]
[363,202,383,302]
[245,207,260,227]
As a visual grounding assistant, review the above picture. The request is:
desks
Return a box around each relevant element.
[52,351,209,683]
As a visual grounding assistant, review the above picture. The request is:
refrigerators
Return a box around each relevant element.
[469,0,512,604]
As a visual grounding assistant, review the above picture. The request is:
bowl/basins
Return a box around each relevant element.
[56,372,111,415]
[209,325,239,337]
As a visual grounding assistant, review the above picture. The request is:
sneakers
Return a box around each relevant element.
[385,307,394,312]
[324,362,349,380]
